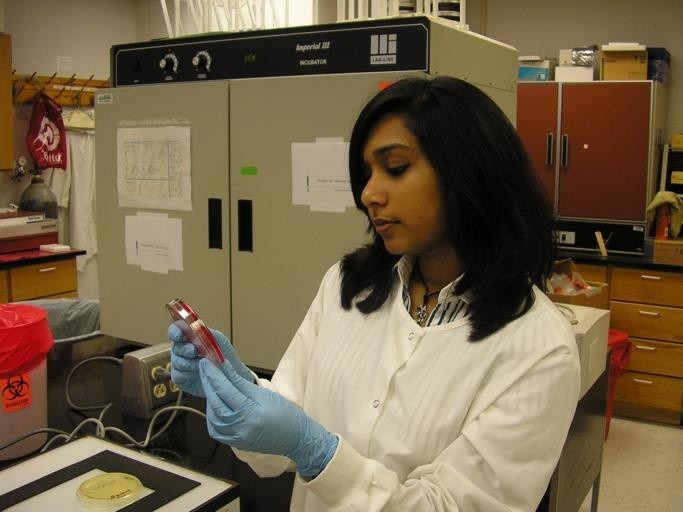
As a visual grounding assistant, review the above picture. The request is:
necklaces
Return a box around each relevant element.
[414,262,441,327]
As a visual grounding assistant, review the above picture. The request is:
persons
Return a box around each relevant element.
[166,75,582,511]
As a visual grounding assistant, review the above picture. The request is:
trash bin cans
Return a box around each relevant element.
[604,327,629,440]
[0,303,56,462]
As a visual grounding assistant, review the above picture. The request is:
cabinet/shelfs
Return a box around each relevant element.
[9,254,78,301]
[608,267,682,433]
[0,267,10,304]
[518,81,669,223]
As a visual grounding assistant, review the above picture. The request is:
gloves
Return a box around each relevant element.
[199,358,338,479]
[168,322,254,398]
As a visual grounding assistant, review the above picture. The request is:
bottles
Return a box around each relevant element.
[654,202,670,240]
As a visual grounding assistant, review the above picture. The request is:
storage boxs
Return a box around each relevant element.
[546,281,608,309]
[600,44,648,81]
